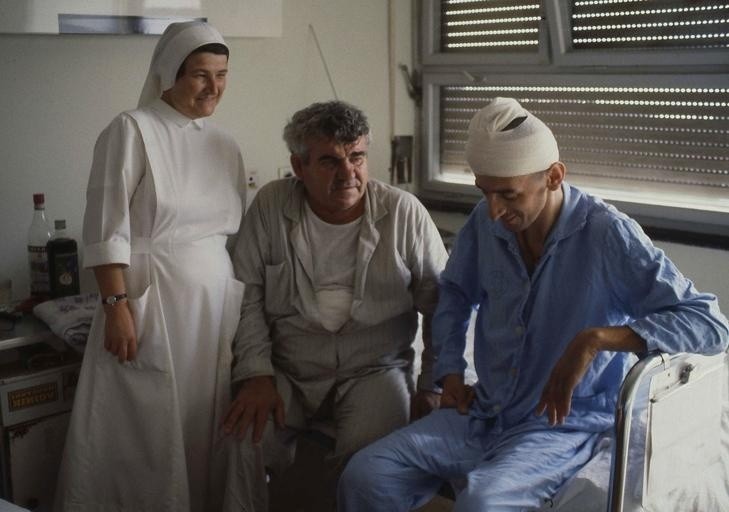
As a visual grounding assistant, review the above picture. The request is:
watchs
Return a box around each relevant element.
[102,294,127,304]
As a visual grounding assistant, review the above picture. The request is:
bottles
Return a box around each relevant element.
[47,220,81,299]
[27,193,53,300]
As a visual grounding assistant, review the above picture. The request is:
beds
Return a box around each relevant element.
[313,301,729,510]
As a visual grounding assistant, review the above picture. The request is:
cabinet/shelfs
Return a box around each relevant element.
[0,311,84,512]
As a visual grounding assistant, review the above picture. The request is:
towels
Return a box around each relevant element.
[33,292,100,345]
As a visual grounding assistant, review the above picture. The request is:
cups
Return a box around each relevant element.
[0,279,14,331]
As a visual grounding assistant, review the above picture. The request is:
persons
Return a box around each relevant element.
[206,100,450,512]
[50,18,270,512]
[334,95,729,512]
